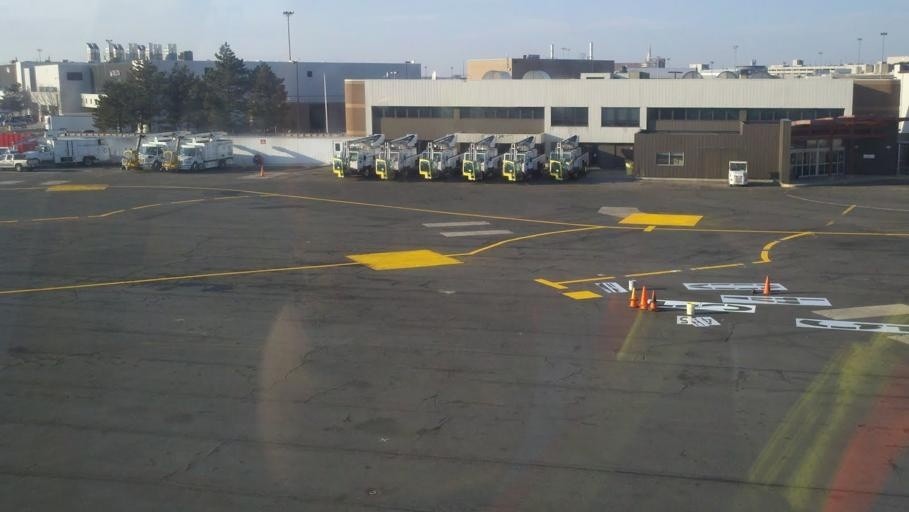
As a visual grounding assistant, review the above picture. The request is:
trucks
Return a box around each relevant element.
[21,135,110,167]
[136,136,234,173]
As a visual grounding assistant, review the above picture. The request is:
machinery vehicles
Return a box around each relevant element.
[332,133,591,182]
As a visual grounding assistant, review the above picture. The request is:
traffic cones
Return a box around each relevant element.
[761,274,772,297]
[629,285,658,312]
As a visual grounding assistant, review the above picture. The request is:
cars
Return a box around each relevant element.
[2,115,33,128]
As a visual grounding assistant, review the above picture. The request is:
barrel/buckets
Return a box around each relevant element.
[687,303,695,315]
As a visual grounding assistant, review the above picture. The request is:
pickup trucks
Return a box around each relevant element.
[0,153,40,172]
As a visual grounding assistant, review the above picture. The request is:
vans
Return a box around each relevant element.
[728,160,749,187]
